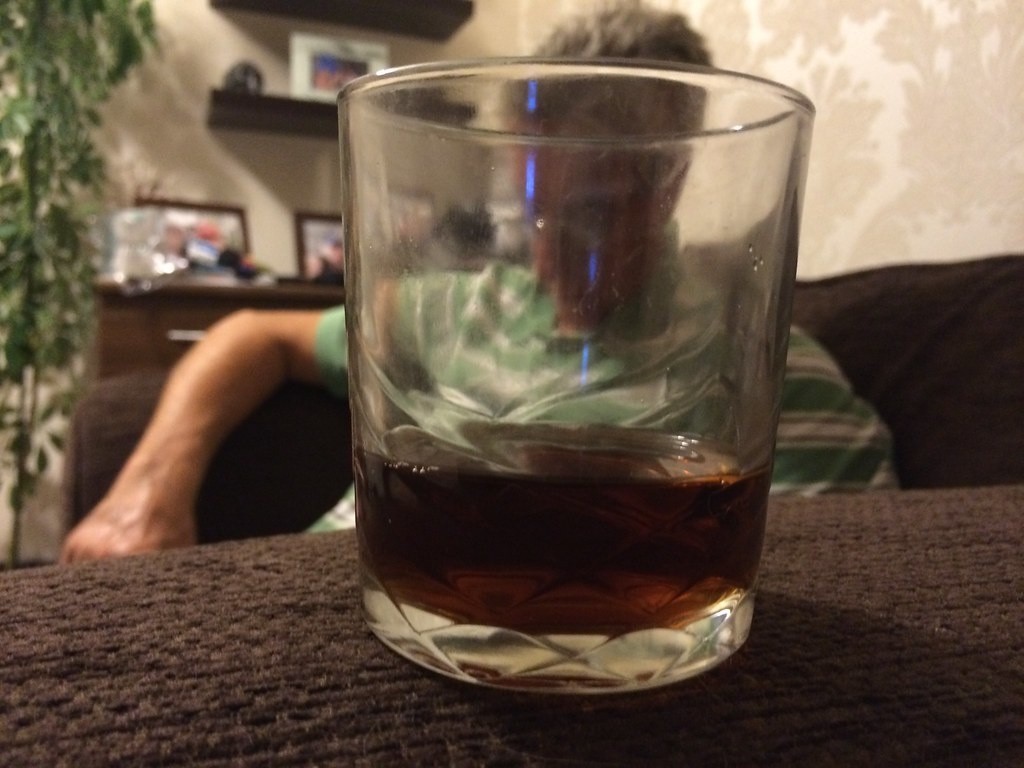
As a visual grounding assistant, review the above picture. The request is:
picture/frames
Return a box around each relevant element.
[137,196,249,255]
[295,211,343,280]
[288,31,391,104]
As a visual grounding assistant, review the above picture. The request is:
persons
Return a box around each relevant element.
[58,1,903,568]
[305,233,345,284]
[163,221,242,269]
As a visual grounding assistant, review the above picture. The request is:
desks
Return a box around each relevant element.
[0,481,1024,768]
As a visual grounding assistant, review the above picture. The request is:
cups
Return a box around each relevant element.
[333,56,816,693]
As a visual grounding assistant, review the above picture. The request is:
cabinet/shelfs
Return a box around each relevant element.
[91,277,348,372]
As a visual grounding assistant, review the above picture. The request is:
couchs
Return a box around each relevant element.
[0,247,1024,545]
